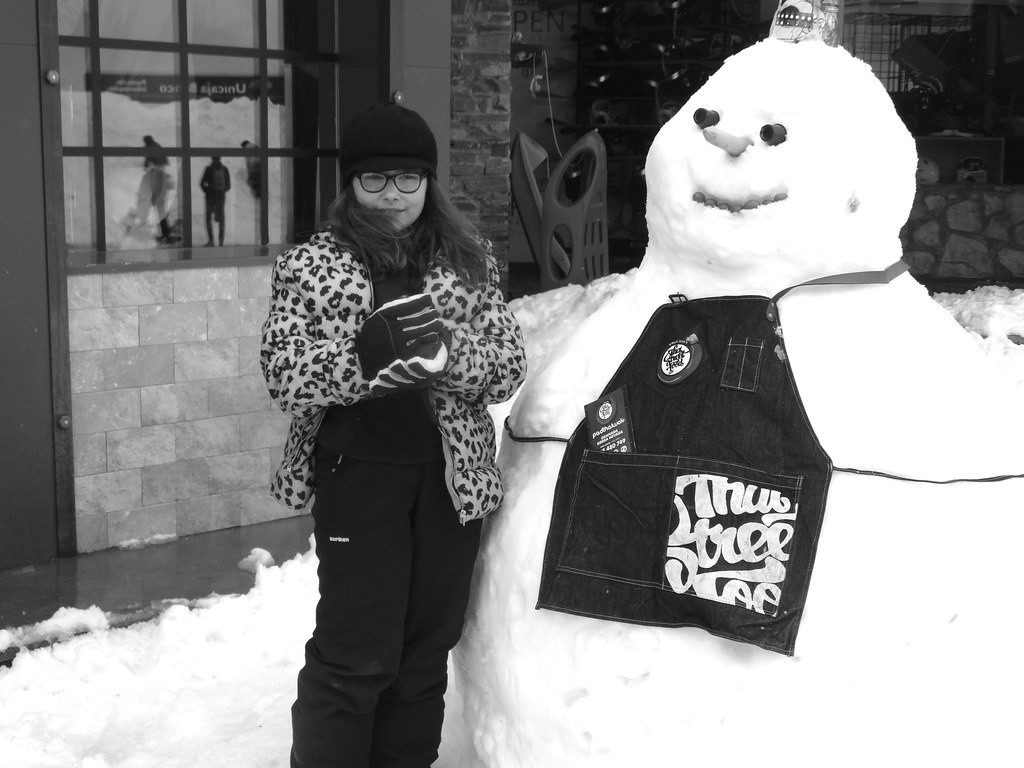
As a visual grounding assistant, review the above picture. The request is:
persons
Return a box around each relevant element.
[260,101,527,768]
[140,134,261,248]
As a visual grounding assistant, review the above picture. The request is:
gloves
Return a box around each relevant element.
[353,294,444,381]
[369,327,453,394]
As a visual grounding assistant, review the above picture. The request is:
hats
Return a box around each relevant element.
[338,98,438,191]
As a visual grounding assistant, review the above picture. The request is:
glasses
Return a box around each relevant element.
[353,170,429,193]
